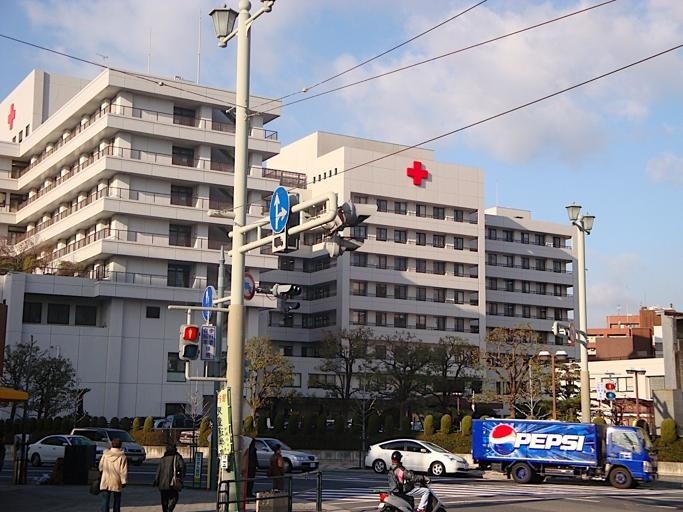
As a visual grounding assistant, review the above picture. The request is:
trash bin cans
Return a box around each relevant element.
[256,490,289,512]
[64,444,98,485]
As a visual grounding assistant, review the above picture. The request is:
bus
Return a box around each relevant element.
[555,374,657,442]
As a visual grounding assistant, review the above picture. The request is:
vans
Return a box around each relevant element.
[69,427,145,466]
[165,414,214,429]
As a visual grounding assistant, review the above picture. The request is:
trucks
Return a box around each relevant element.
[470,417,658,489]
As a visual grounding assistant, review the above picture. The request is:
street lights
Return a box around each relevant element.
[626,369,646,420]
[565,201,595,424]
[538,350,568,420]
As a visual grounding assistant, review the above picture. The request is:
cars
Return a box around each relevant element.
[362,438,468,477]
[252,437,319,472]
[24,434,96,467]
[179,430,213,446]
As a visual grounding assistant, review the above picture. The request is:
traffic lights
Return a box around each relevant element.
[320,204,371,258]
[178,324,199,361]
[604,381,616,401]
[277,285,301,315]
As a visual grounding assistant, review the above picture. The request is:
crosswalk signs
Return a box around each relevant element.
[201,286,216,321]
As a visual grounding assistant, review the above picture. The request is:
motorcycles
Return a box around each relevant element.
[376,470,448,511]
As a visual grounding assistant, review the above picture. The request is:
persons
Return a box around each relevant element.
[98,438,127,511]
[241,438,261,501]
[150,442,186,511]
[269,443,284,490]
[388,450,431,511]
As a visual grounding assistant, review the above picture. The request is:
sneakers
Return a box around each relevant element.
[415,507,426,512]
[246,494,256,497]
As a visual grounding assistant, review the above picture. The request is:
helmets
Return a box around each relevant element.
[391,451,402,460]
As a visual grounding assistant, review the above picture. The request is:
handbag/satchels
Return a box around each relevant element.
[169,476,184,492]
[88,477,100,496]
[266,468,271,477]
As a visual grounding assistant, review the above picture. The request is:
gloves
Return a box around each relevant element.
[121,483,126,488]
[424,476,431,484]
